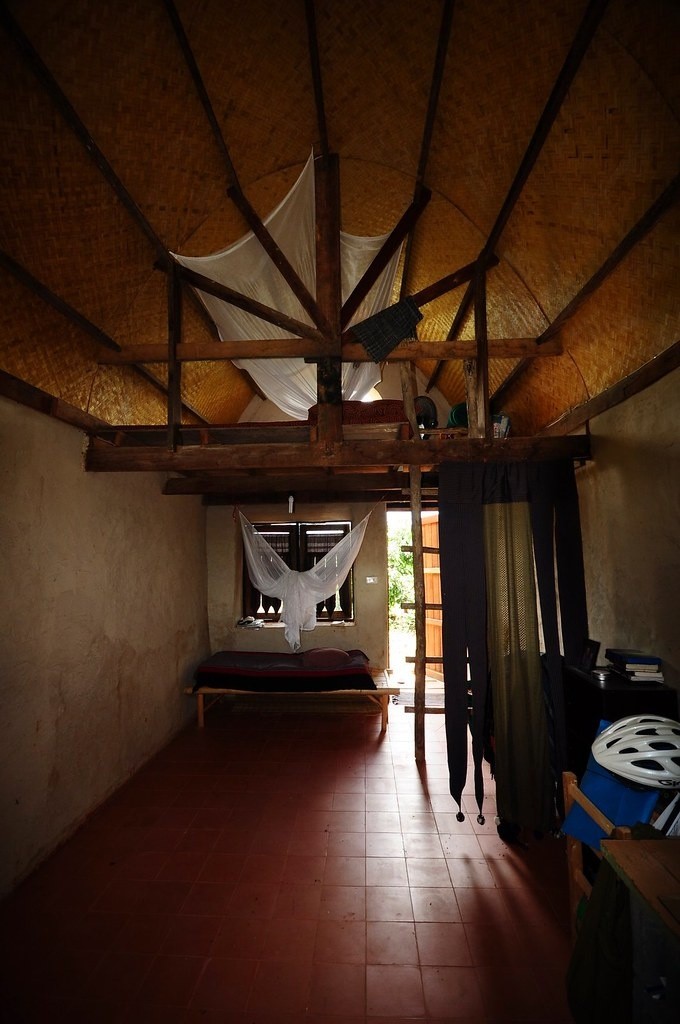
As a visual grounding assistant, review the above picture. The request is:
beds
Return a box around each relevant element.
[113,421,414,445]
[185,669,400,727]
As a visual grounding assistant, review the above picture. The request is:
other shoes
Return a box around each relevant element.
[236,616,265,628]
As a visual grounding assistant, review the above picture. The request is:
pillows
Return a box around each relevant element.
[302,647,352,667]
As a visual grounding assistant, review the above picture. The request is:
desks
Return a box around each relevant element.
[597,839,680,1024]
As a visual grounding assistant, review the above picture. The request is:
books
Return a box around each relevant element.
[605,648,664,686]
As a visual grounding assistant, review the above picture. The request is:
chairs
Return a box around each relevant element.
[562,771,633,940]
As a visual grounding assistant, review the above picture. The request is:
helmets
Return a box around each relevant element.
[590,712,680,791]
